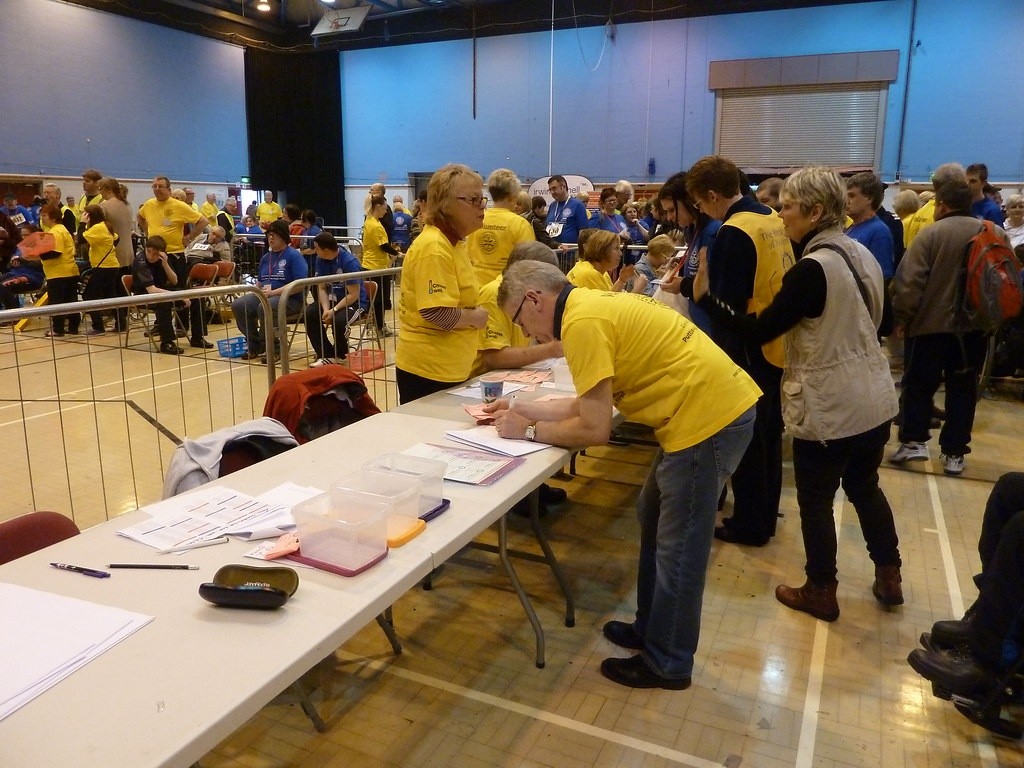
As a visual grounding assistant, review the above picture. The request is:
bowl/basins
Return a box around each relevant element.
[291,452,449,571]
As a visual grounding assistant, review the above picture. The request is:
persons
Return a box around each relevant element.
[0,169,428,355]
[460,154,1024,548]
[908,471,1024,701]
[395,162,489,406]
[303,232,368,368]
[482,260,765,690]
[231,219,308,364]
[692,166,904,621]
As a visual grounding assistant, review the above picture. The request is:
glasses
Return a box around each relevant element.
[604,199,617,204]
[692,199,703,210]
[266,233,279,239]
[511,290,542,328]
[660,252,671,261]
[547,184,562,193]
[455,196,488,208]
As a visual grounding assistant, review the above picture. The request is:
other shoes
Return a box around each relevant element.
[529,483,567,506]
[44,324,214,354]
[376,323,399,339]
[510,496,548,520]
[895,382,901,387]
[980,376,993,401]
[608,433,627,445]
[714,516,776,547]
[242,343,265,360]
[889,355,904,373]
[892,405,947,429]
[261,352,280,364]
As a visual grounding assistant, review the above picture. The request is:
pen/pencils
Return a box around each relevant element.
[105,564,200,570]
[156,536,229,554]
[661,253,678,265]
[50,562,111,579]
[509,395,518,410]
[634,269,640,278]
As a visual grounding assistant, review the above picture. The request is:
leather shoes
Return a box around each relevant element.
[601,653,693,691]
[873,564,905,605]
[908,647,1024,698]
[931,602,1006,657]
[603,620,647,650]
[775,578,840,622]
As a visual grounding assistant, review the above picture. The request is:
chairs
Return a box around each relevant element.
[255,286,310,362]
[297,394,431,629]
[12,236,236,352]
[219,441,401,733]
[315,281,382,358]
[0,511,81,565]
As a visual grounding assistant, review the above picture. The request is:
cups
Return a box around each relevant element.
[480,375,504,404]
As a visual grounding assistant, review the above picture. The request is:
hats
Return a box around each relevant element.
[982,182,1002,193]
[265,219,292,244]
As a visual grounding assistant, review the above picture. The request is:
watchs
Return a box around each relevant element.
[525,419,539,441]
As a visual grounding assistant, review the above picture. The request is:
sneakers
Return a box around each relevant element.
[888,441,929,466]
[309,358,347,367]
[942,454,967,474]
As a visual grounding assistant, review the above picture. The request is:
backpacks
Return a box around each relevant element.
[953,220,1024,337]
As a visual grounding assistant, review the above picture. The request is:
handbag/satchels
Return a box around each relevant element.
[652,268,693,324]
[83,275,91,283]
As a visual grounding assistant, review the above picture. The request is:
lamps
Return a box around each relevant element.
[256,0,271,13]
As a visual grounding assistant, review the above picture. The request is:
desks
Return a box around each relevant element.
[0,356,621,768]
